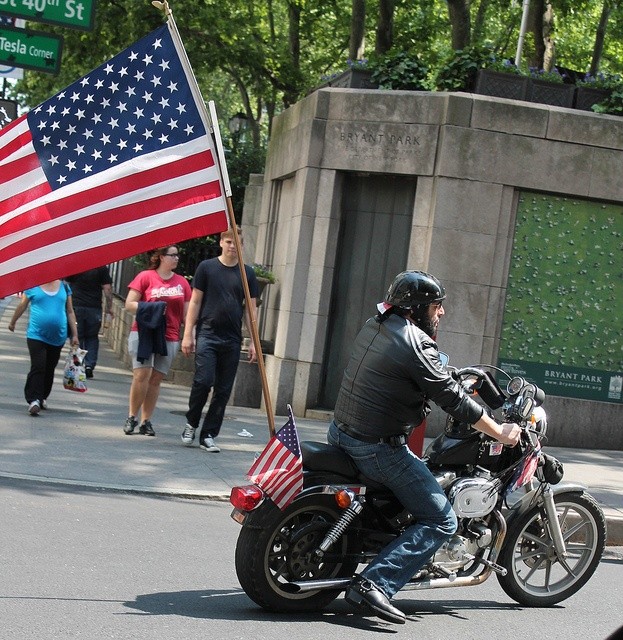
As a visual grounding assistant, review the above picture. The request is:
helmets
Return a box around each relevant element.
[384,270,447,306]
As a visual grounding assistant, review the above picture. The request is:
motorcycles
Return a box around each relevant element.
[228,351,607,612]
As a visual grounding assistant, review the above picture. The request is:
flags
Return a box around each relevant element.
[246,418,305,511]
[0,19,231,299]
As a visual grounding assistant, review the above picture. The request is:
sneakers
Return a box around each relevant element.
[181,423,196,444]
[200,434,220,452]
[28,400,40,415]
[139,420,156,435]
[123,417,138,434]
[41,400,46,408]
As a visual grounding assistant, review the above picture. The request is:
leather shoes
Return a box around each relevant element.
[85,366,92,377]
[345,576,406,624]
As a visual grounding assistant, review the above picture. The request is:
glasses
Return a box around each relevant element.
[161,252,180,257]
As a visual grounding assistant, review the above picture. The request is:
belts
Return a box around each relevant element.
[333,417,408,447]
[73,303,100,308]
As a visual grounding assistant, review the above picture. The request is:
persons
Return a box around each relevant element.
[124,243,191,435]
[181,229,259,452]
[61,267,113,379]
[326,271,524,625]
[8,280,79,414]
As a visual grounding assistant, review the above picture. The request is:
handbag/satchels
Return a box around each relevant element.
[63,345,88,392]
